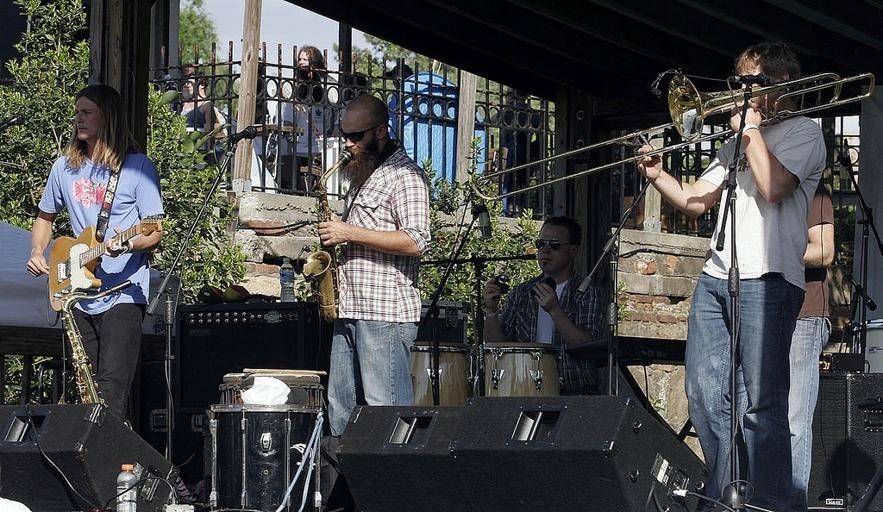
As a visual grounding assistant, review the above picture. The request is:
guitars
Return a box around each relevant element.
[49,214,161,313]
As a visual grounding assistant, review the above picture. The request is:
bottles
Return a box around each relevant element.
[279,258,296,303]
[115,463,138,512]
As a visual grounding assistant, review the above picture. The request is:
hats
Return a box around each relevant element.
[386,65,412,78]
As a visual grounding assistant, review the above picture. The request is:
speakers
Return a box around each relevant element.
[1,401,194,512]
[810,370,882,512]
[336,393,716,512]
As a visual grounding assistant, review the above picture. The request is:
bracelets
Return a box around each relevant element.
[30,246,43,257]
[741,124,757,136]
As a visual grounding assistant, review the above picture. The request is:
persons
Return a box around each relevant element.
[786,184,836,510]
[481,214,605,396]
[636,40,827,512]
[386,64,489,201]
[316,96,434,437]
[26,84,166,424]
[489,85,542,218]
[275,45,338,196]
[180,72,229,183]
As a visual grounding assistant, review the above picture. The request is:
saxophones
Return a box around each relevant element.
[59,282,132,414]
[303,149,353,322]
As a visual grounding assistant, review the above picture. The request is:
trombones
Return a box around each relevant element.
[470,72,874,201]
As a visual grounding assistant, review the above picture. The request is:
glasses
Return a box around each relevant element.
[534,239,570,250]
[339,124,379,142]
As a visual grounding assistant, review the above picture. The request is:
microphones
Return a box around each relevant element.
[476,196,495,241]
[485,251,539,266]
[652,128,673,142]
[847,273,878,318]
[732,72,774,88]
[229,125,258,146]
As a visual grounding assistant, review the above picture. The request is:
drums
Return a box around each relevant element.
[409,342,469,408]
[482,343,560,399]
[219,374,324,412]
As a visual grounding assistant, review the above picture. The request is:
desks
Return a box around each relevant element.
[255,122,304,136]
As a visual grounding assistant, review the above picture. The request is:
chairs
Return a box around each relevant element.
[301,155,322,196]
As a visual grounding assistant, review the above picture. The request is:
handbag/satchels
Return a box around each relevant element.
[204,123,226,142]
